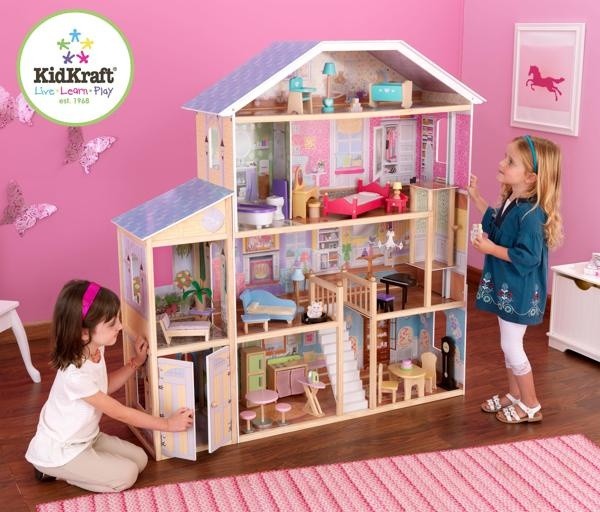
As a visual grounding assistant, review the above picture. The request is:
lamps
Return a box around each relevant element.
[0,300,42,385]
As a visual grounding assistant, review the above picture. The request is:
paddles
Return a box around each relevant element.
[35,433,600,512]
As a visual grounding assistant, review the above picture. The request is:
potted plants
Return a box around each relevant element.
[511,21,586,137]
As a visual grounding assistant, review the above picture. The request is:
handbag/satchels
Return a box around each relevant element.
[127,360,139,370]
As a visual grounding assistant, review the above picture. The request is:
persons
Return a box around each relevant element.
[26,279,193,494]
[470,137,562,424]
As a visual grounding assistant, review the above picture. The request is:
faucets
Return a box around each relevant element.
[496,399,544,424]
[480,392,521,413]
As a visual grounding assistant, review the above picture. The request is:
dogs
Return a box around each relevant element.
[526,64,565,101]
